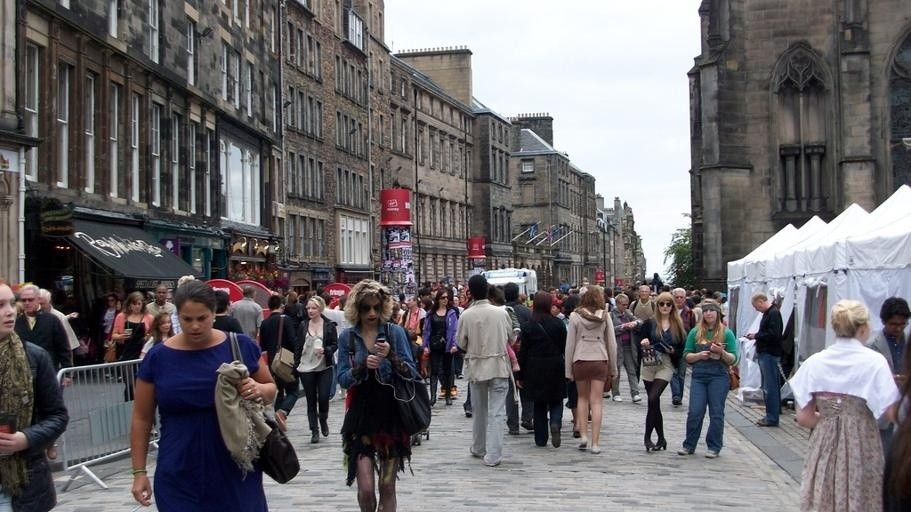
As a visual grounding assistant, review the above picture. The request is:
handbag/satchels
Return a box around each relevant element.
[392,373,430,435]
[729,366,740,389]
[641,345,663,368]
[103,340,117,362]
[262,412,299,484]
[271,348,296,383]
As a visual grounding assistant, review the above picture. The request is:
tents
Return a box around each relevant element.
[727,182,911,405]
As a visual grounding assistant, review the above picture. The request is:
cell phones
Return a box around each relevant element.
[376,337,386,344]
[711,343,721,353]
[744,333,751,339]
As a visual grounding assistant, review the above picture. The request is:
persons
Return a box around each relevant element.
[883,329,911,512]
[866,296,911,457]
[787,298,903,512]
[1,276,428,512]
[421,277,783,466]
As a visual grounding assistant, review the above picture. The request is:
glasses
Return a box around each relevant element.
[656,302,671,307]
[22,298,34,302]
[886,319,909,326]
[360,304,381,313]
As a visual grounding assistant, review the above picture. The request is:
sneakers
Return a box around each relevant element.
[274,409,287,431]
[430,386,610,467]
[612,396,720,458]
[311,430,319,443]
[320,419,328,436]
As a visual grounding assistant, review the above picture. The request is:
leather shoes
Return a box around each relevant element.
[47,444,57,459]
[757,415,778,426]
